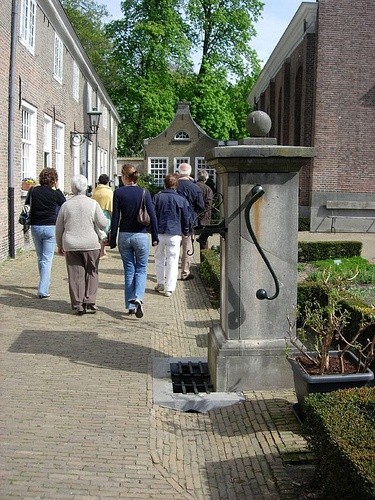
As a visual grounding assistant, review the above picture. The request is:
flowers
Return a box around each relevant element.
[22,177,36,184]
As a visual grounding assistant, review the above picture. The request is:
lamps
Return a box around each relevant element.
[70,107,102,147]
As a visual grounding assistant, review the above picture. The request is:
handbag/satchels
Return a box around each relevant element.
[19,205,31,226]
[137,189,150,227]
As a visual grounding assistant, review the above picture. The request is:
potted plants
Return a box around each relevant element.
[283,256,375,415]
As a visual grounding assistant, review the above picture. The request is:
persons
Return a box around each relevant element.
[177,162,204,280]
[109,164,160,318]
[25,168,66,297]
[55,175,109,314]
[195,170,213,225]
[153,175,190,297]
[93,174,113,259]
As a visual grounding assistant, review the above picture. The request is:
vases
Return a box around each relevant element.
[22,182,36,191]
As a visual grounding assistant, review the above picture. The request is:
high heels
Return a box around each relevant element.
[78,310,86,316]
[129,308,136,316]
[134,298,144,318]
[87,304,99,310]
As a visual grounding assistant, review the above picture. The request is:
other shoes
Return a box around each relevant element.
[40,294,50,298]
[154,284,164,291]
[181,274,194,280]
[165,291,172,297]
